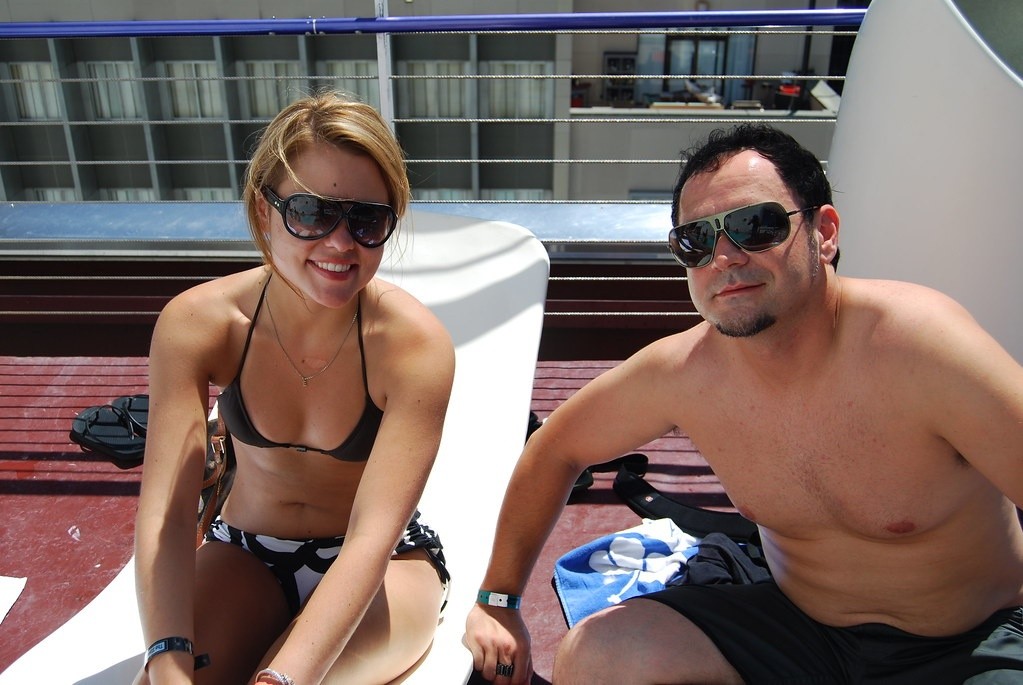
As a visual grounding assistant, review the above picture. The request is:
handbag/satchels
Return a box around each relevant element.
[200,418,237,522]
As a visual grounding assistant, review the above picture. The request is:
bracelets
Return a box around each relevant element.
[254,667,295,685]
[475,588,523,611]
[143,635,196,673]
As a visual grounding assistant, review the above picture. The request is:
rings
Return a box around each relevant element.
[496,662,515,678]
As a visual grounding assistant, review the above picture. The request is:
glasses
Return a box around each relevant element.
[260,184,398,248]
[667,202,820,268]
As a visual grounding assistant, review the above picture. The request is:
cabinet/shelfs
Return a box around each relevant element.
[603,52,637,106]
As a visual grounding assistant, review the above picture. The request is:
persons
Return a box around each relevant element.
[133,89,455,685]
[464,119,1023,685]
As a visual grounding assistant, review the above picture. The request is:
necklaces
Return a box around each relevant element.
[263,286,359,389]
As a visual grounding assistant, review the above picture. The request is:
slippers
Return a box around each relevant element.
[113,394,150,435]
[72,405,146,461]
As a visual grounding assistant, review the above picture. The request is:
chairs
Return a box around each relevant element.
[0,213,551,685]
